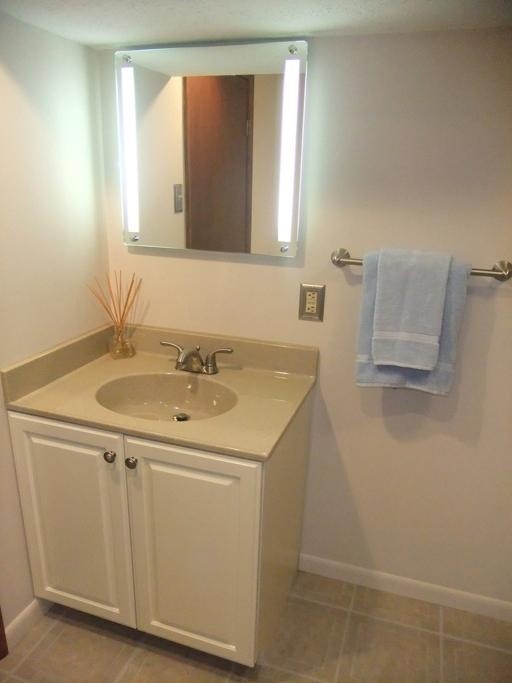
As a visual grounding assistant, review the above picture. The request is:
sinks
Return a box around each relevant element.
[95,373,239,423]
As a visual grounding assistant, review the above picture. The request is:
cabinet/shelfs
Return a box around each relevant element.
[9,379,318,667]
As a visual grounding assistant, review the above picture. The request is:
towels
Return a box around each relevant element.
[350,248,473,397]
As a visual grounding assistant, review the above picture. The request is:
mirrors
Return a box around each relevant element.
[110,36,309,265]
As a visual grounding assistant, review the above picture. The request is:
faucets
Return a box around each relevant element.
[176,347,210,375]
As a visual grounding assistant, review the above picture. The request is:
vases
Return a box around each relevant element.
[107,322,135,358]
